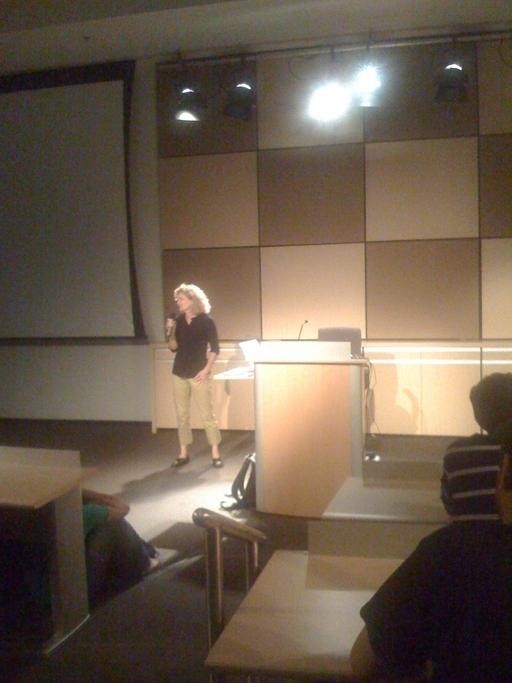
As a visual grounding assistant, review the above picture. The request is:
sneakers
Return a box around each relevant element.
[170,456,224,469]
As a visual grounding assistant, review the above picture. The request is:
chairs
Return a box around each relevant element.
[316,326,364,360]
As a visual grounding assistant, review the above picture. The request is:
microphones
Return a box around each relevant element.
[298,320,308,341]
[166,312,176,336]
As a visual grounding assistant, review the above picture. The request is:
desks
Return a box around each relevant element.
[0,456,93,662]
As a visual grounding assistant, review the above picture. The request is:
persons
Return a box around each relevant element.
[164,283,224,468]
[1,489,159,681]
[346,433,511,683]
[439,372,512,518]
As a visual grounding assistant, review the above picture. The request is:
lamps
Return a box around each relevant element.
[425,37,471,102]
[170,51,258,123]
